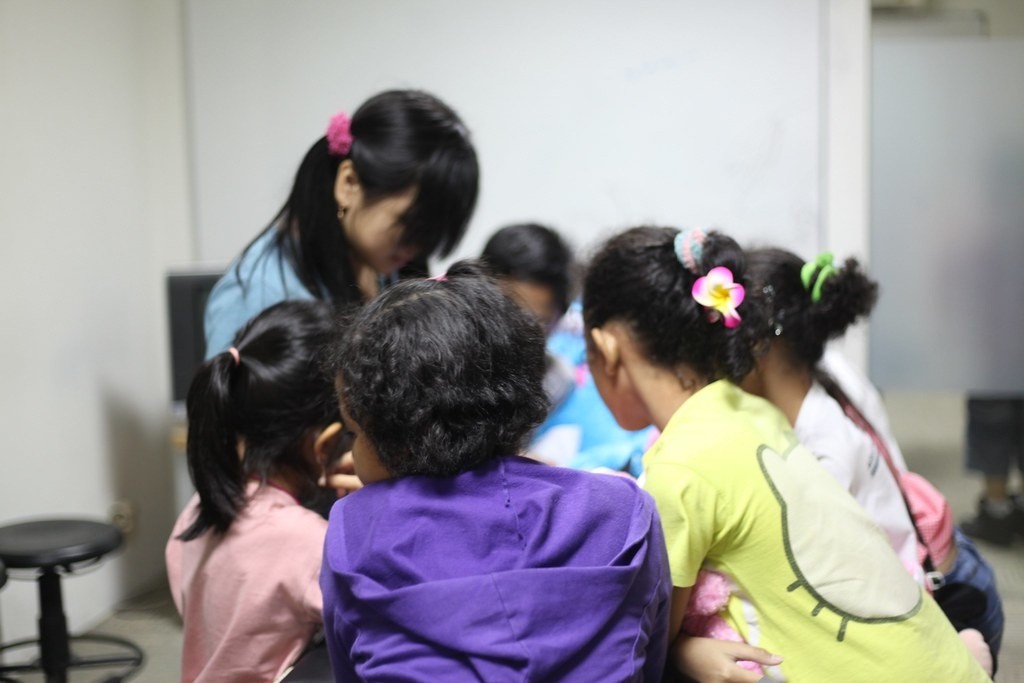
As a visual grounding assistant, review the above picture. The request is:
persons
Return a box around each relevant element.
[165,90,1006,683]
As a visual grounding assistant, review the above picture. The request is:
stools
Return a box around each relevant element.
[0,519,145,683]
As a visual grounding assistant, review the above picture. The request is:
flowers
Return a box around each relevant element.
[692,267,747,329]
